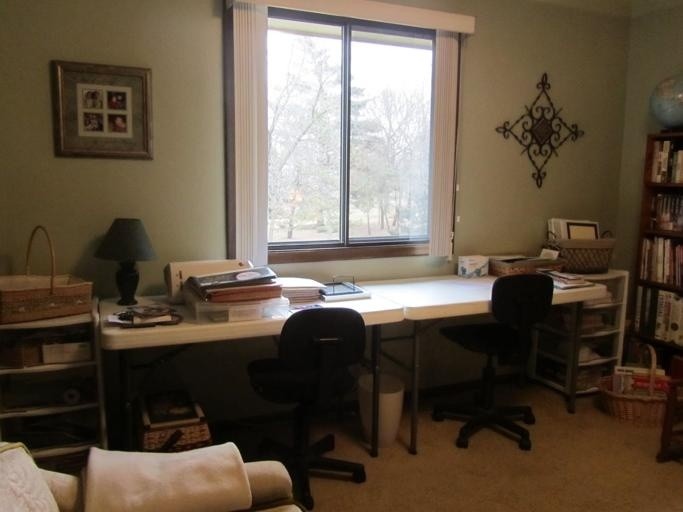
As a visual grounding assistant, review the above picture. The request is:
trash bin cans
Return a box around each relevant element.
[358,374,404,448]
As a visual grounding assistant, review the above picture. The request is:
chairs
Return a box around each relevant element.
[426,274,555,451]
[241,306,370,509]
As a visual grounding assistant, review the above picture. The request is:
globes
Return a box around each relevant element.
[650,75,683,134]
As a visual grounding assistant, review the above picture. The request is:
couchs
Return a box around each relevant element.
[0,438,308,511]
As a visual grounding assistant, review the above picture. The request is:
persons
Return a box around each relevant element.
[81,89,128,134]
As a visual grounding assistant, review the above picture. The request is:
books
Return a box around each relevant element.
[186,276,282,303]
[628,134,683,361]
[191,265,278,291]
[547,271,585,285]
[608,364,671,399]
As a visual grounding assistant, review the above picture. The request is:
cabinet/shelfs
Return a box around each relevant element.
[621,129,682,382]
[2,296,108,470]
[525,267,630,416]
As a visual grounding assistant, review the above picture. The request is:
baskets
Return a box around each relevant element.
[489,258,567,275]
[546,228,615,272]
[138,396,212,453]
[0,225,92,324]
[600,346,667,422]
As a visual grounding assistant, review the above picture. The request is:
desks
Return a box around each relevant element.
[94,281,407,460]
[353,269,610,456]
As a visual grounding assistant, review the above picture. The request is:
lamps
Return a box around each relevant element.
[93,213,159,308]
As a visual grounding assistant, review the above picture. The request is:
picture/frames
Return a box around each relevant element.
[46,55,154,161]
